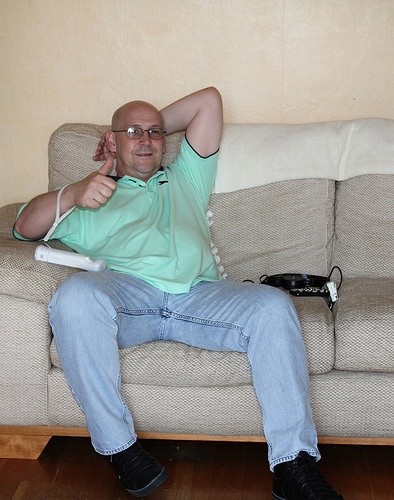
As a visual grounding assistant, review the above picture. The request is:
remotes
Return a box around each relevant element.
[289,287,330,297]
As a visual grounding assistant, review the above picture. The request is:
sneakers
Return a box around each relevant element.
[108,442,167,498]
[271,452,344,500]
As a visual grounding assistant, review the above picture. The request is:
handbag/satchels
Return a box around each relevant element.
[241,266,343,309]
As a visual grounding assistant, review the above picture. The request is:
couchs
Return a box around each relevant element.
[0,118,394,460]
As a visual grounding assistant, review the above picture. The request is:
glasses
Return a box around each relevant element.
[111,126,166,140]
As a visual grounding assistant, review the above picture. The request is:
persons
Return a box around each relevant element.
[12,85,344,500]
